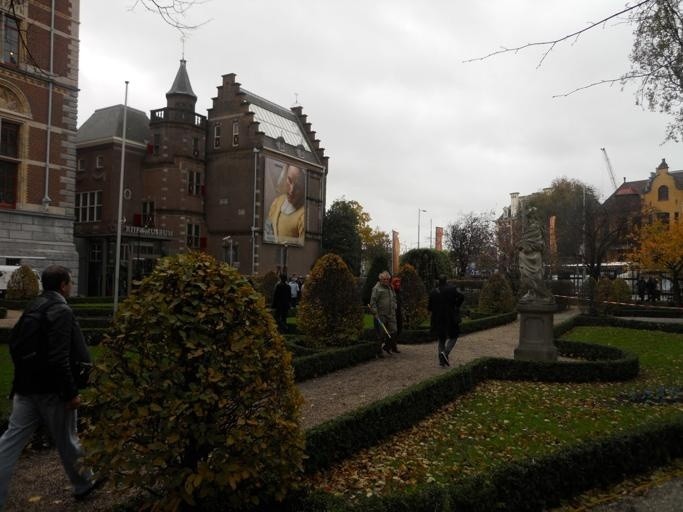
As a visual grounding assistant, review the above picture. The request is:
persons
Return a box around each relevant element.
[270,263,312,331]
[263,165,305,247]
[370,270,398,359]
[391,277,401,354]
[636,277,660,302]
[427,273,464,368]
[0,265,106,508]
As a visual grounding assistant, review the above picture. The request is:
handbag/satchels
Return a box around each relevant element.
[9,299,59,385]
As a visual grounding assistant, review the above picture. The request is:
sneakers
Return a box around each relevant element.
[378,345,400,358]
[439,351,450,368]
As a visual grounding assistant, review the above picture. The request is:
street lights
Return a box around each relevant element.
[221,234,233,268]
[415,206,427,249]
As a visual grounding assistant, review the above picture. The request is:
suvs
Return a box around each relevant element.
[0,261,40,298]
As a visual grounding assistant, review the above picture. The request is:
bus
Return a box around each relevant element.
[543,262,637,296]
